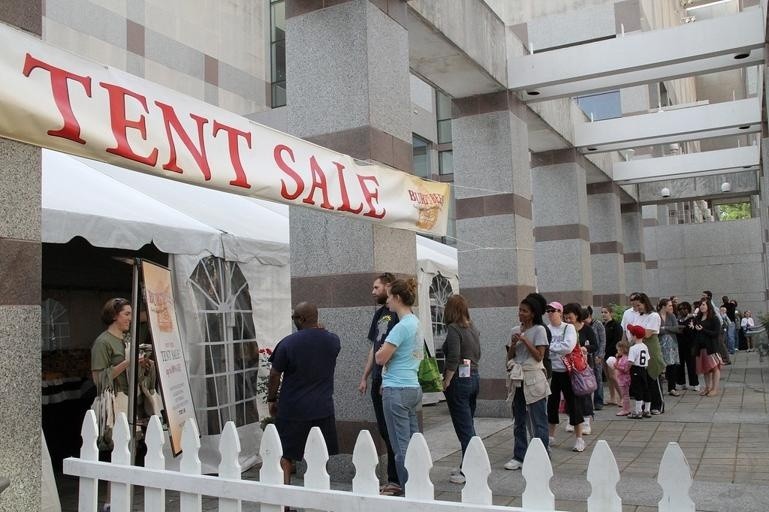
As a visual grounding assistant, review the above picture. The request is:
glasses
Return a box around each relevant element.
[546,310,557,312]
[292,315,306,322]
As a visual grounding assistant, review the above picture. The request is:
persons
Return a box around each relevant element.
[560,302,592,436]
[581,290,755,418]
[266,301,340,512]
[525,291,554,459]
[375,278,425,496]
[137,358,157,469]
[442,294,482,483]
[544,301,587,452]
[91,297,154,504]
[503,292,554,470]
[356,272,413,487]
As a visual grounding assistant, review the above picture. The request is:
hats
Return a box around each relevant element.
[627,323,645,338]
[547,301,564,312]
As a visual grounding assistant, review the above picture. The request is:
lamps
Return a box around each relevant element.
[587,112,597,150]
[656,50,751,198]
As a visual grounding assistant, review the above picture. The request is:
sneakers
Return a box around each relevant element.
[503,459,523,469]
[548,415,591,452]
[595,400,664,418]
[669,384,720,397]
[379,483,403,496]
[447,464,465,483]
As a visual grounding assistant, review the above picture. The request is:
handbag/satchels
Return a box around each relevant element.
[90,366,130,452]
[560,341,598,396]
[418,338,444,392]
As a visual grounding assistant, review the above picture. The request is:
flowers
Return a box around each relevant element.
[254,348,282,427]
